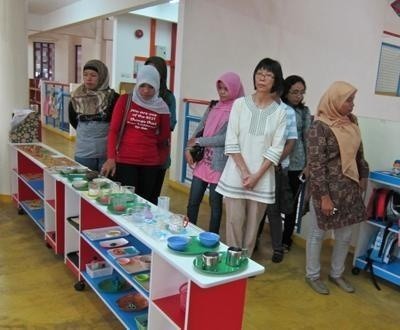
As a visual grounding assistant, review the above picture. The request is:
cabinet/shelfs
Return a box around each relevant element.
[9,138,106,257]
[350,170,399,289]
[61,179,265,330]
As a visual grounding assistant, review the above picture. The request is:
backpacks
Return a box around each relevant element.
[363,187,400,265]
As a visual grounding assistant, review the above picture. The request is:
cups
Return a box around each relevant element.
[199,250,223,269]
[168,213,189,232]
[226,247,248,267]
[157,195,171,215]
[126,205,147,227]
[100,182,135,210]
[87,182,100,197]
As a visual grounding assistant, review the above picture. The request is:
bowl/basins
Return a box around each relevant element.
[167,235,191,251]
[92,177,108,186]
[72,178,88,188]
[199,232,221,247]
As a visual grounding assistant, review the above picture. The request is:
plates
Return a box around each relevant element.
[73,179,111,192]
[169,236,221,256]
[82,225,152,313]
[96,193,137,205]
[106,202,151,216]
[194,251,250,274]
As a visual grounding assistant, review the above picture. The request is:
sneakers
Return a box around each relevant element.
[272,244,355,294]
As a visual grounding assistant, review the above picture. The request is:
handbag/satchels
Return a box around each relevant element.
[191,128,204,162]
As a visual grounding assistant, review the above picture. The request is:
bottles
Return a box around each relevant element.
[392,160,400,176]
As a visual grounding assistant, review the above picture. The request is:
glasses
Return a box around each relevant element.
[289,89,305,96]
[256,72,275,81]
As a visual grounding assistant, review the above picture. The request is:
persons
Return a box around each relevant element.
[185,73,246,235]
[213,57,288,259]
[253,75,300,264]
[68,59,120,171]
[281,75,311,252]
[100,64,170,205]
[143,56,177,199]
[304,79,368,294]
